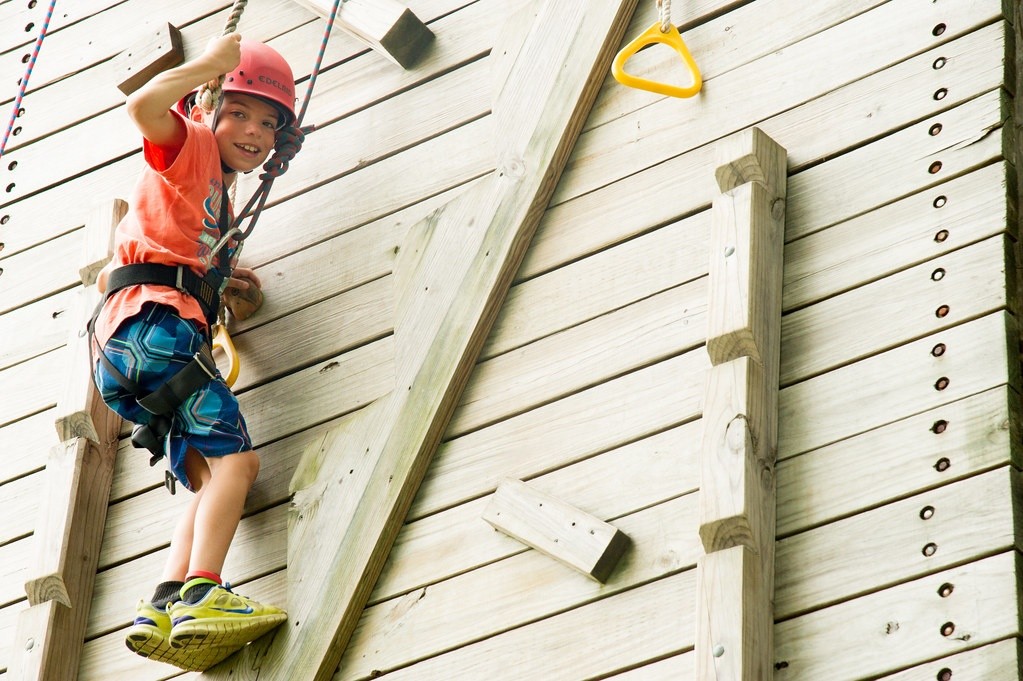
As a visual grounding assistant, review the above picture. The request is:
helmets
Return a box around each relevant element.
[177,40,296,147]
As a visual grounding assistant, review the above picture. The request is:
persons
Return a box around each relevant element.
[90,34,296,670]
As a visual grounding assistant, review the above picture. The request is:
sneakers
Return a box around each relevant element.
[125,600,247,671]
[166,582,287,650]
